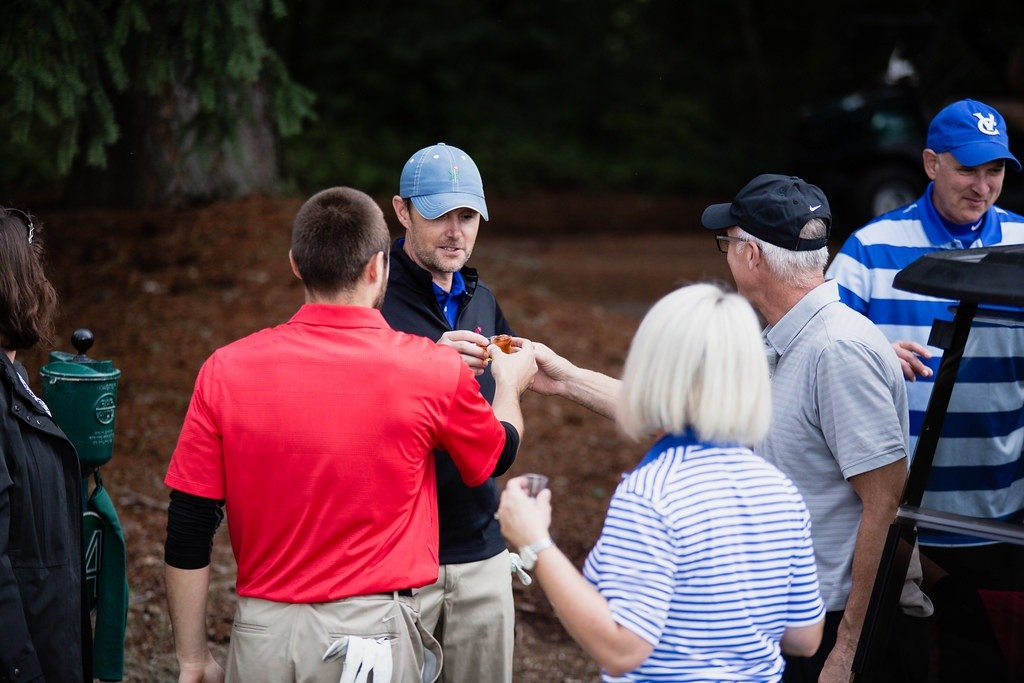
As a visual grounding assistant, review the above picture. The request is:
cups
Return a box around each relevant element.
[526,473,549,499]
[476,335,512,362]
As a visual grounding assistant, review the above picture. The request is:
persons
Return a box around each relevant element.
[825,99,1023,683]
[511,176,933,683]
[498,285,827,682]
[162,187,539,683]
[379,143,515,683]
[0,208,92,683]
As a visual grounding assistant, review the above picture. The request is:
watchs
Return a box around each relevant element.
[518,539,555,571]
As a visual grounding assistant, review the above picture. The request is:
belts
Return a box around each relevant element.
[376,587,413,597]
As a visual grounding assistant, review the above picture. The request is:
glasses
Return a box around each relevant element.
[716,232,761,253]
[6,209,36,251]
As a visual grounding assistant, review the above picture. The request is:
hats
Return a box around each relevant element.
[926,98,1022,171]
[400,143,489,221]
[700,173,831,252]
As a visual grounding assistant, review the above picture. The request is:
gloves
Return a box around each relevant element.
[510,552,532,585]
[323,637,392,683]
